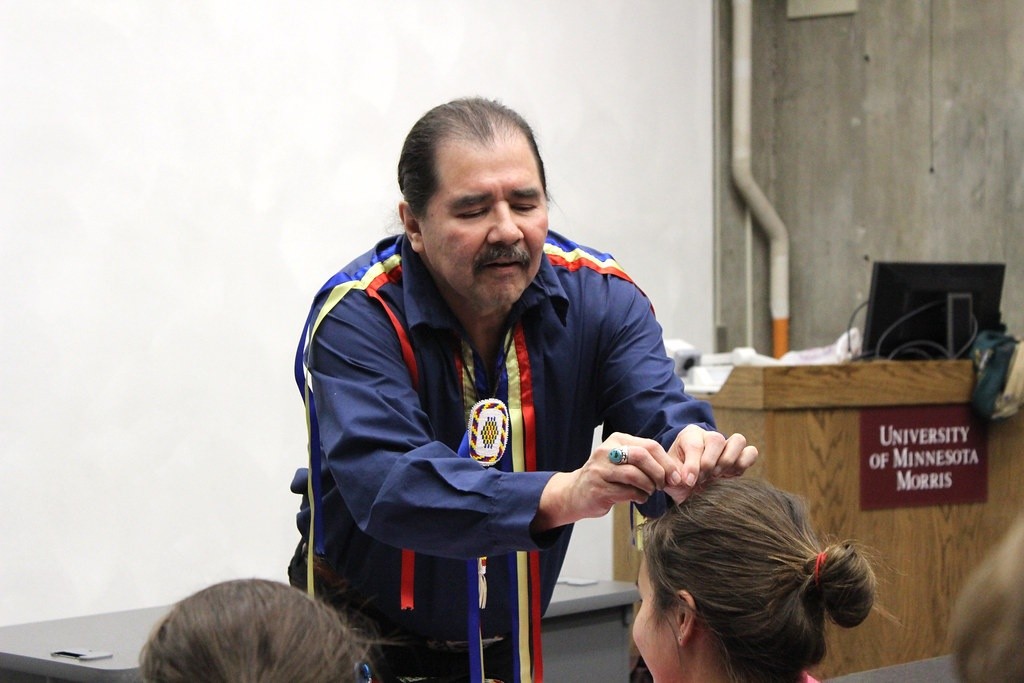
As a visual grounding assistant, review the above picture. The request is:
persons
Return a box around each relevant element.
[286,96,759,683]
[139,578,377,683]
[632,474,876,683]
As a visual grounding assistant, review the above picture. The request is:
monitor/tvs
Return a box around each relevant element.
[862,261,1006,359]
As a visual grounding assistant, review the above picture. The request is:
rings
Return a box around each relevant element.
[609,446,629,465]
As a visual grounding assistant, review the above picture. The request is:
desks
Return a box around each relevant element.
[0,578,640,683]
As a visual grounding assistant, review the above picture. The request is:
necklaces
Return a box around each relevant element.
[458,324,517,468]
